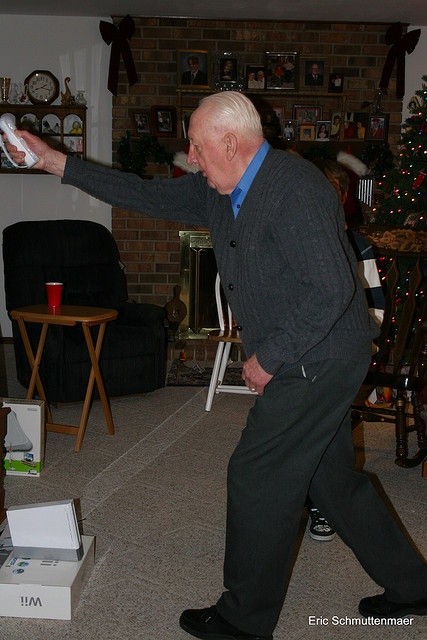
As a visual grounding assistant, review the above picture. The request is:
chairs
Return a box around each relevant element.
[206,270,258,412]
[350,251,426,467]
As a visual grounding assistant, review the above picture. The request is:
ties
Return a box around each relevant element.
[313,73,317,80]
[191,74,194,84]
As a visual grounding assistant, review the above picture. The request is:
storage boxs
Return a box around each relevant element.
[0,398,45,478]
[0,534,96,620]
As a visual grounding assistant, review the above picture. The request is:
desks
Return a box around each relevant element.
[355,223,427,408]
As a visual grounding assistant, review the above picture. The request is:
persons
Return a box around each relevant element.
[317,123,329,140]
[219,60,234,81]
[306,62,322,84]
[140,116,149,133]
[266,60,273,84]
[283,123,294,138]
[264,113,275,127]
[11,90,426,639]
[282,57,294,82]
[305,142,387,540]
[272,65,284,86]
[247,71,260,90]
[180,56,207,86]
[332,115,342,141]
[304,128,311,137]
[355,120,365,137]
[255,70,265,88]
[344,119,352,137]
[371,117,382,139]
[332,72,342,88]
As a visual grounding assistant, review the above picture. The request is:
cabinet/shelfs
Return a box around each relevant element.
[1,105,87,174]
[176,89,346,142]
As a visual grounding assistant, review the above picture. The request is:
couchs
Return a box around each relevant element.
[3,219,167,405]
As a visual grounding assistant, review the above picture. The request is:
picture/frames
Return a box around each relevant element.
[366,114,390,141]
[329,110,368,141]
[327,69,345,92]
[177,107,196,140]
[128,108,152,136]
[177,48,210,89]
[152,105,177,138]
[316,121,331,142]
[292,104,323,128]
[300,125,315,141]
[265,52,299,91]
[262,105,284,138]
[244,63,265,91]
[282,119,298,139]
[212,49,244,91]
[297,55,328,95]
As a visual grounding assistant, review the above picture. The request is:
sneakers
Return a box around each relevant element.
[358,594,427,622]
[179,603,274,640]
[307,493,338,543]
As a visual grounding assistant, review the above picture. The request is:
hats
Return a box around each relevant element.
[372,118,380,122]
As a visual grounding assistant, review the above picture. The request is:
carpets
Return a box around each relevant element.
[168,360,246,387]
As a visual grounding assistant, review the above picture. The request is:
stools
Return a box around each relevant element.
[11,304,118,453]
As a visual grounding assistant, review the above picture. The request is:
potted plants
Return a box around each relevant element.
[117,130,174,179]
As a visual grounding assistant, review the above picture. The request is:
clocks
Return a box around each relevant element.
[24,70,59,105]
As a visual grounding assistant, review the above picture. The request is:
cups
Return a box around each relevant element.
[0,76,10,106]
[45,282,63,312]
